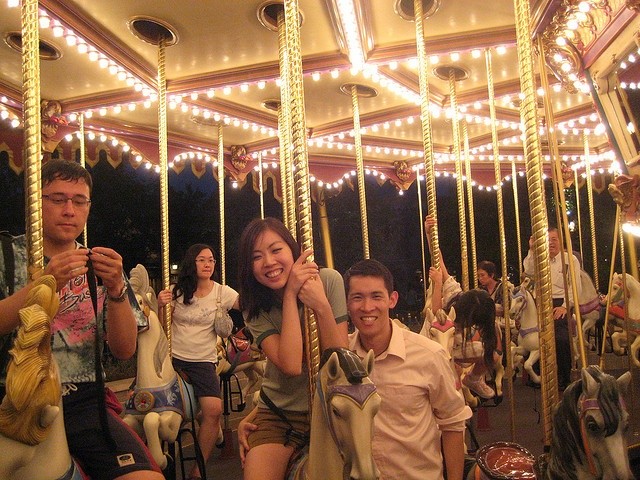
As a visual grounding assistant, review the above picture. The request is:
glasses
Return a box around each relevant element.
[42,193,92,208]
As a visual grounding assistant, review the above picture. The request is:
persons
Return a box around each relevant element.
[523,227,583,390]
[477,261,510,316]
[238,216,348,480]
[420,214,496,399]
[158,243,241,480]
[237,257,473,480]
[0,159,166,480]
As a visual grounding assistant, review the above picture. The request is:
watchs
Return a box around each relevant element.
[109,283,129,301]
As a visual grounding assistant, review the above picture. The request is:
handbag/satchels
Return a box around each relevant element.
[213,284,234,338]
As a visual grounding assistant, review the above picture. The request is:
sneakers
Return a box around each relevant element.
[462,373,496,401]
